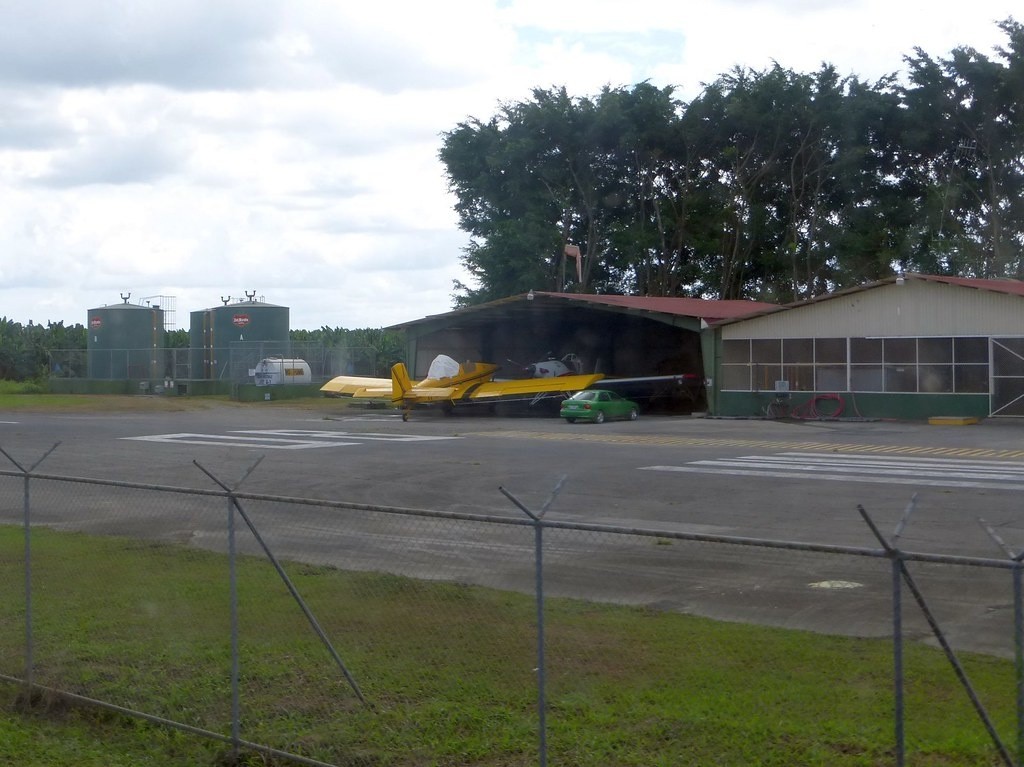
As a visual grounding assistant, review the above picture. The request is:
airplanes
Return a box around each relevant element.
[319,353,606,421]
[489,351,697,404]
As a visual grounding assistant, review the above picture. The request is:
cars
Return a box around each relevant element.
[560,390,639,424]
